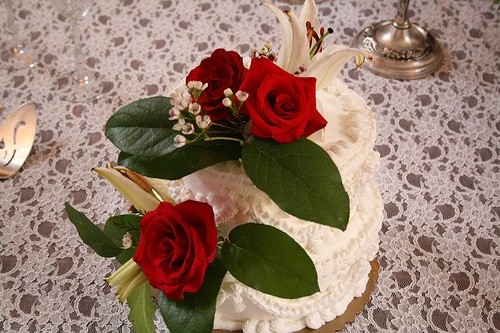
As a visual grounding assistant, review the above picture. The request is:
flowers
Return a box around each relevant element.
[63,164,321,332]
[104,48,351,231]
